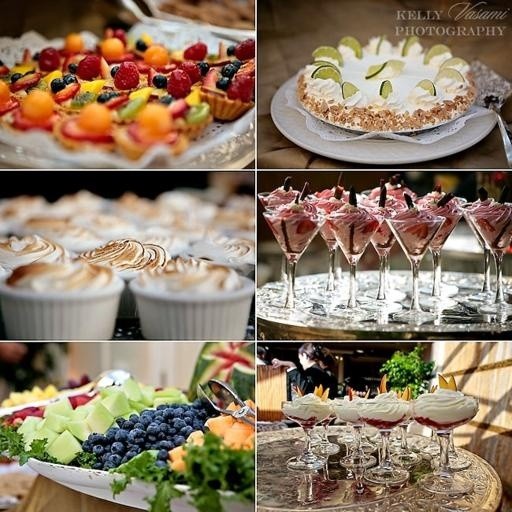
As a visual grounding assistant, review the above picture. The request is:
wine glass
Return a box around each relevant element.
[261,189,512,328]
[283,400,475,495]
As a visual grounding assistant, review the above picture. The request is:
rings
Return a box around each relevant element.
[274,360,278,365]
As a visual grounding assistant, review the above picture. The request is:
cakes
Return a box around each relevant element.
[280,372,479,436]
[0,186,254,341]
[262,173,511,261]
[0,26,255,169]
[295,35,479,134]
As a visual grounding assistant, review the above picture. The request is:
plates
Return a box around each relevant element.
[271,66,501,167]
[16,402,254,512]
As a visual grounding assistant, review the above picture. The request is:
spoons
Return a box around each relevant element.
[476,76,512,163]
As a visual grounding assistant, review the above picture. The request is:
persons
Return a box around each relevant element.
[256,344,270,366]
[0,342,29,405]
[317,345,339,398]
[270,341,332,402]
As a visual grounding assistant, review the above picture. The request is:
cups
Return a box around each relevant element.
[1,260,252,340]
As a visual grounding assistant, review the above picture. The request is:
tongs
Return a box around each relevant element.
[196,374,251,431]
